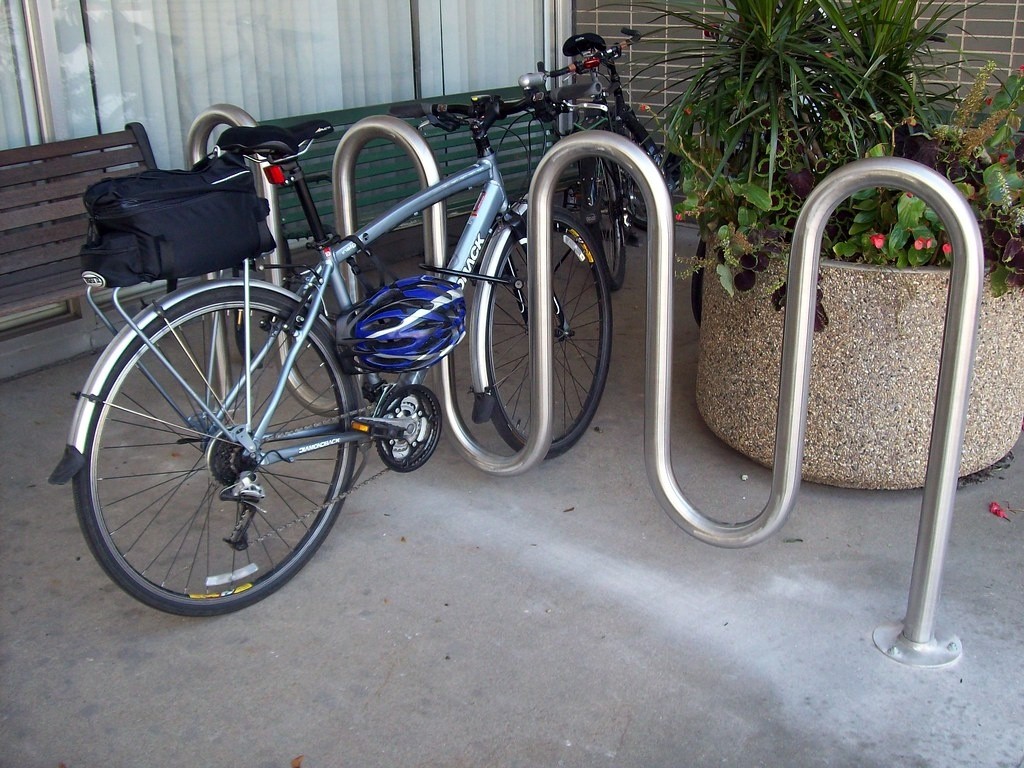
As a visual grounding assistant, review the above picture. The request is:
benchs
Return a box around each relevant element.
[0,123,231,415]
[234,87,587,284]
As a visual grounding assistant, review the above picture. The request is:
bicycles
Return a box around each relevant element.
[535,26,685,295]
[45,74,610,618]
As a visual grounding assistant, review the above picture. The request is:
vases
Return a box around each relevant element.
[694,235,1024,490]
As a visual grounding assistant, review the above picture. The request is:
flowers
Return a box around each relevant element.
[582,0,1024,277]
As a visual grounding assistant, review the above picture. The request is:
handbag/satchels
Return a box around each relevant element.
[80,145,277,288]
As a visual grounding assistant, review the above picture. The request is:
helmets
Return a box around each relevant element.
[336,275,467,375]
